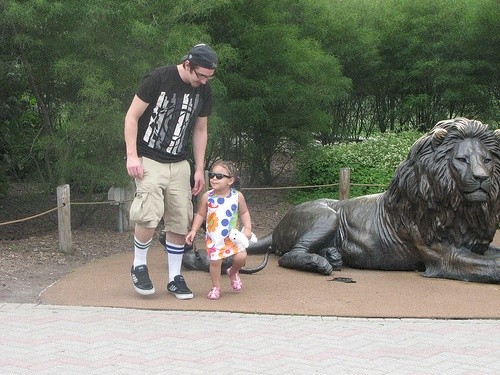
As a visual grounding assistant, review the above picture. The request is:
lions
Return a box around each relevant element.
[195,117,500,285]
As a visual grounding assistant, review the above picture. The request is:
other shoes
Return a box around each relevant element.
[227,266,242,291]
[207,286,221,299]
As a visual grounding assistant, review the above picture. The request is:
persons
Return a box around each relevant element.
[123,43,218,300]
[185,159,252,300]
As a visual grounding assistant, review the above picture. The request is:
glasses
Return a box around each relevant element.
[208,173,231,179]
[191,67,215,80]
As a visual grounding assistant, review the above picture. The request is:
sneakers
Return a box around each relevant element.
[167,275,193,300]
[131,264,155,295]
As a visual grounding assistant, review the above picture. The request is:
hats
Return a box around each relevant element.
[182,43,218,69]
